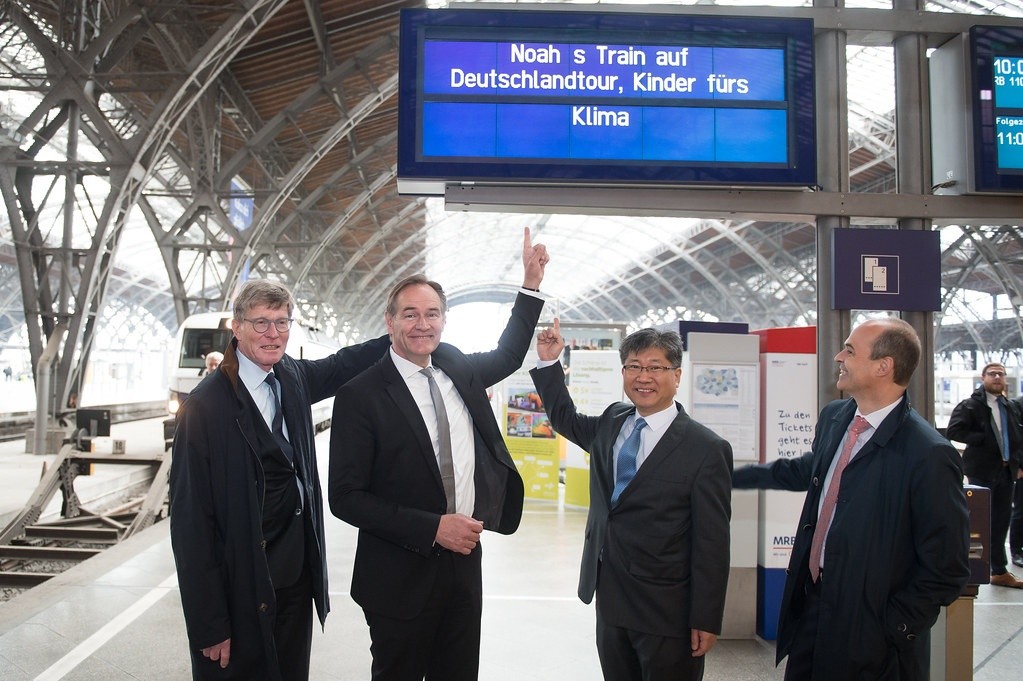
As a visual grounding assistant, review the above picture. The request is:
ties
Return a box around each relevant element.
[809,416,872,584]
[264,371,293,466]
[420,368,456,513]
[610,418,648,505]
[996,397,1009,460]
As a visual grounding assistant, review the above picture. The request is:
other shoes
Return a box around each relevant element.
[1012,554,1023,568]
[989,570,1023,589]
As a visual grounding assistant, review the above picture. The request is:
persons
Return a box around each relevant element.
[201,350,225,373]
[167,279,390,681]
[528,318,735,681]
[732,318,971,681]
[947,363,1023,587]
[328,227,551,681]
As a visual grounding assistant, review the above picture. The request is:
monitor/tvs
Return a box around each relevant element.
[395,6,817,197]
[968,25,1023,193]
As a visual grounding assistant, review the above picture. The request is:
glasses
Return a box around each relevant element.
[241,317,295,333]
[624,364,677,375]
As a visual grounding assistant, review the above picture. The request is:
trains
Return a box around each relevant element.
[162,310,340,453]
